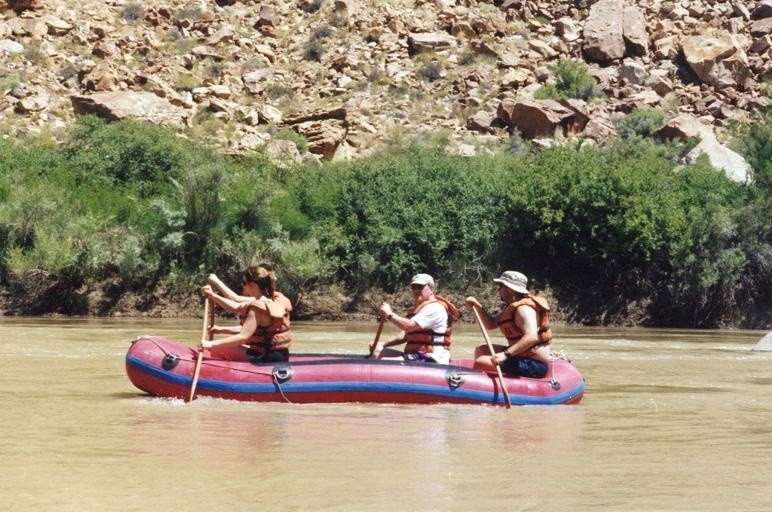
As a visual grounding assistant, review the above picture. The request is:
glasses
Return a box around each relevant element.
[242,280,247,287]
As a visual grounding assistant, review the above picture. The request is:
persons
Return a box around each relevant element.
[464,270,553,379]
[198,260,293,363]
[369,273,460,365]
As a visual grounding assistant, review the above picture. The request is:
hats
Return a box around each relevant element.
[410,272,435,289]
[492,270,530,297]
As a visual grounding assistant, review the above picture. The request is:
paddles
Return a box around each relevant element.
[472,306,510,407]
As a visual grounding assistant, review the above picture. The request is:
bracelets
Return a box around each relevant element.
[388,313,394,320]
[503,350,513,358]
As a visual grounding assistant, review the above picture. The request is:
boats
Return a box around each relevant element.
[126,335,585,404]
